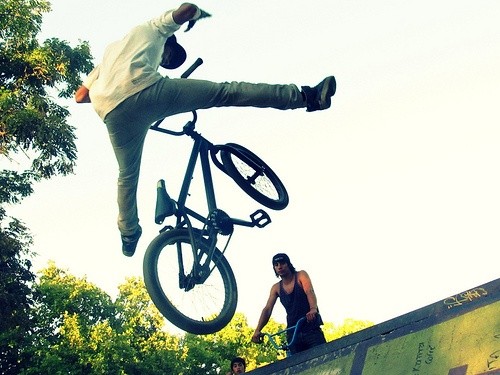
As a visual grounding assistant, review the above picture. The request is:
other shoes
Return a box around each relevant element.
[122,226,142,256]
[299,75,336,113]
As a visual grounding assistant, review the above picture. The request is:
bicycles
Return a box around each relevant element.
[143,58,290,335]
[259,314,320,358]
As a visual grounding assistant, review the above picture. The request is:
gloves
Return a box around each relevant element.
[185,8,212,32]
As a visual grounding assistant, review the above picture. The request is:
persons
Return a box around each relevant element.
[251,253,327,356]
[74,2,337,257]
[227,358,246,375]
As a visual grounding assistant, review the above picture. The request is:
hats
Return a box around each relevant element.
[160,34,187,70]
[272,253,290,265]
